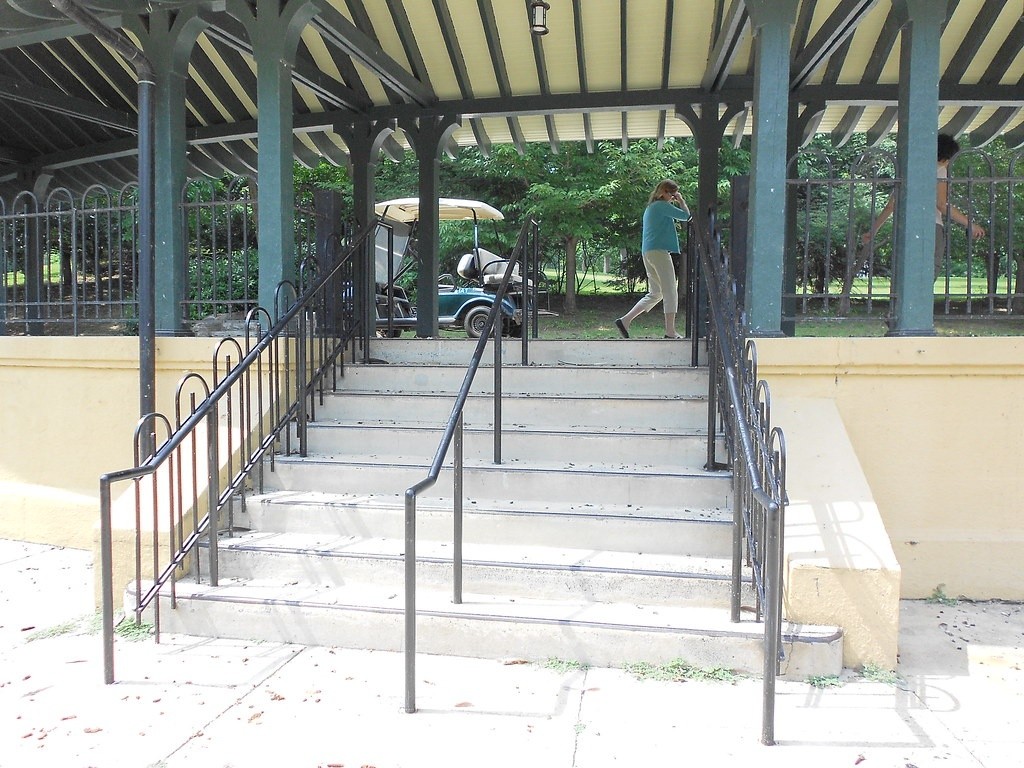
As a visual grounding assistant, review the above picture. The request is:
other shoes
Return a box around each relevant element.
[614,318,629,338]
[665,334,683,339]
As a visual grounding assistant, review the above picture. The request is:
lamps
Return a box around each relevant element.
[529,0,551,37]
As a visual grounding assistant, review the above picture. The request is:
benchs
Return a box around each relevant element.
[472,248,550,291]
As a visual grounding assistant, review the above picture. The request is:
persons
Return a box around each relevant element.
[860,133,986,280]
[614,179,691,338]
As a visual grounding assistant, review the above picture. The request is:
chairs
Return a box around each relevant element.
[437,253,478,291]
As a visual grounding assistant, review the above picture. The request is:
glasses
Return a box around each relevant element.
[668,192,675,199]
